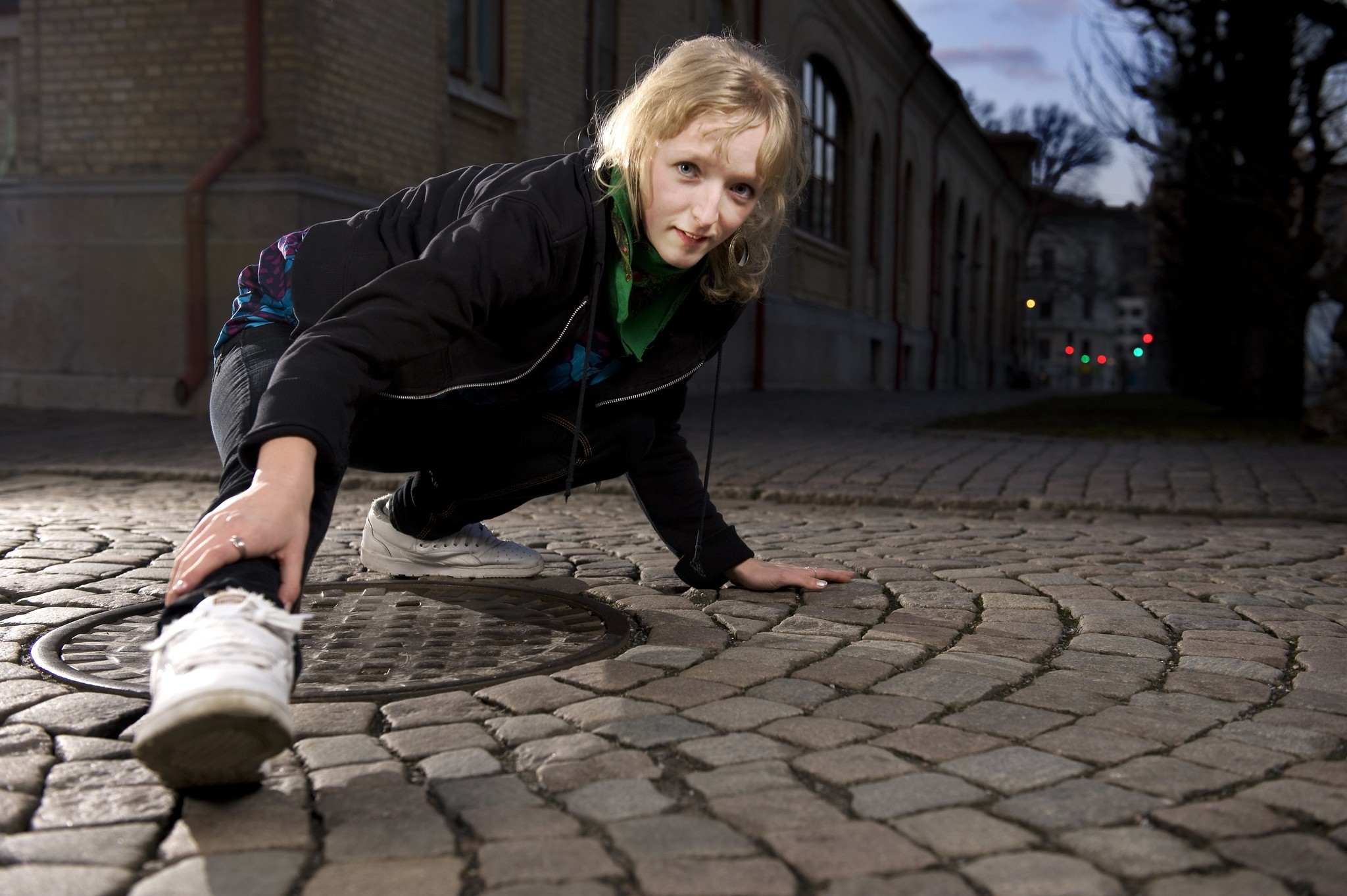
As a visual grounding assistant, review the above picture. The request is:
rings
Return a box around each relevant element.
[228,535,248,559]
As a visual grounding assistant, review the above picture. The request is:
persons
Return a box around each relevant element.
[128,34,855,793]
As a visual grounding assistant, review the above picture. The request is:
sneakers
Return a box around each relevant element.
[359,485,543,579]
[129,585,305,796]
